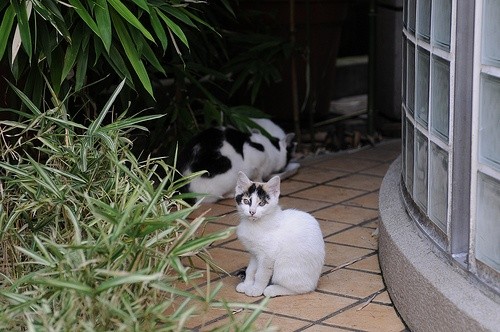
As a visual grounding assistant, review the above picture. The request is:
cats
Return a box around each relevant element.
[162,117,300,204]
[234,172,326,296]
